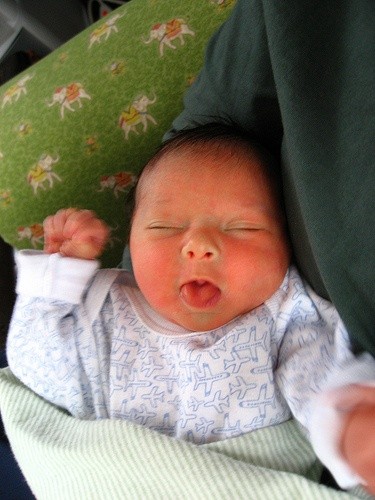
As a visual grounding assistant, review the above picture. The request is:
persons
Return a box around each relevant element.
[120,0,375,489]
[5,119,375,500]
